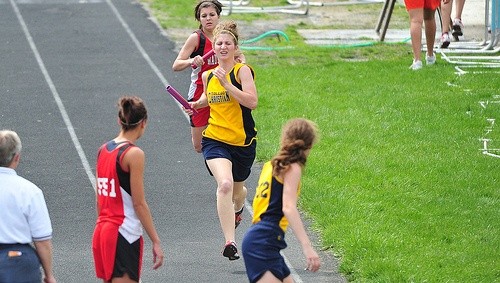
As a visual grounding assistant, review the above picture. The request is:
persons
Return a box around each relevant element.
[173,0,245,153]
[182,20,258,260]
[92,96,164,283]
[0,129,57,283]
[241,118,320,283]
[404,0,466,71]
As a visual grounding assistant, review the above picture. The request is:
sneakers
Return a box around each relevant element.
[220,241,240,261]
[451,19,464,36]
[425,51,437,66]
[234,204,245,229]
[407,58,423,72]
[439,34,450,48]
